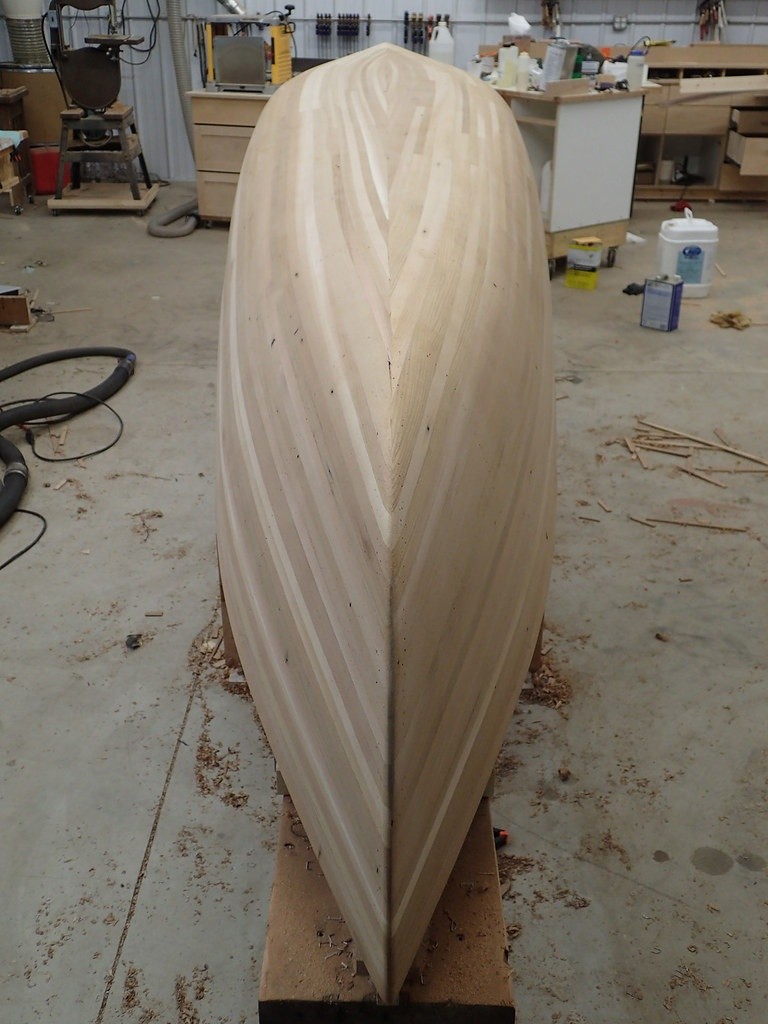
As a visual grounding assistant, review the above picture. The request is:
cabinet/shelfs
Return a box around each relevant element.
[494,80,662,236]
[190,83,279,227]
[625,43,768,203]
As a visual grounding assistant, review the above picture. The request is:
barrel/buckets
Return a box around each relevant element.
[658,207,720,299]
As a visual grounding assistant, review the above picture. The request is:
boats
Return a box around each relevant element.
[213,44,551,1001]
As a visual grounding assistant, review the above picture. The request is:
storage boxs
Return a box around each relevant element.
[478,34,584,91]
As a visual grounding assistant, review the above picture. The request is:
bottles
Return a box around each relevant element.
[496,40,519,87]
[627,50,645,90]
[428,22,454,66]
[517,52,529,92]
[571,48,584,78]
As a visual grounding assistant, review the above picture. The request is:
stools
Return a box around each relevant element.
[55,111,153,200]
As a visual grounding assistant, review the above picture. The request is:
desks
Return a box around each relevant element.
[0,130,30,210]
